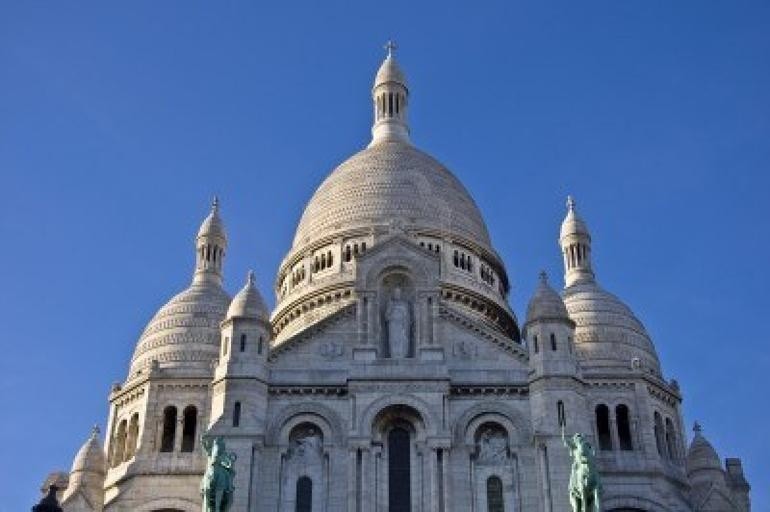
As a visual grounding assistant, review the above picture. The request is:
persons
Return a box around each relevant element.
[481,429,492,459]
[200,437,235,494]
[561,422,605,495]
[296,429,322,464]
[384,288,411,358]
